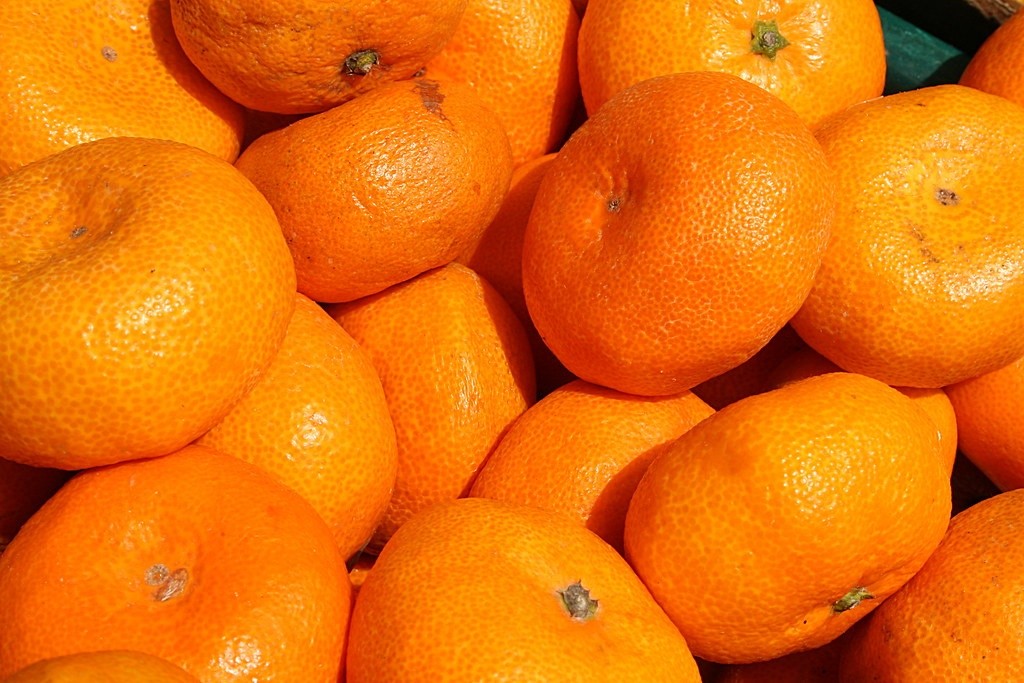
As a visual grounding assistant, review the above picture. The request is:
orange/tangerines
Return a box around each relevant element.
[0,0,1024,683]
